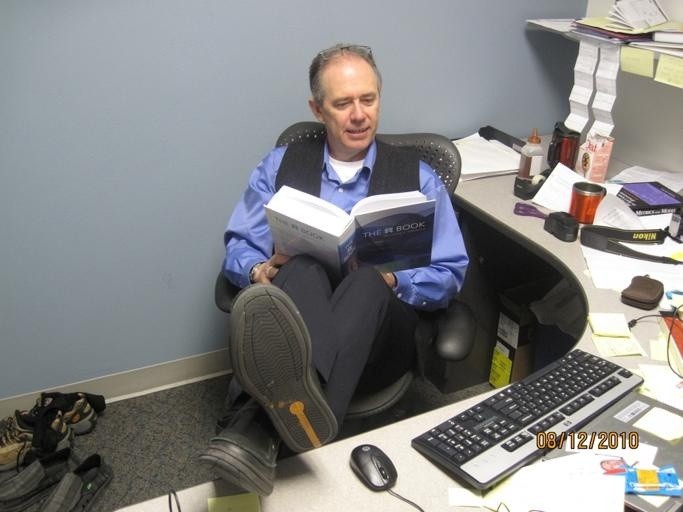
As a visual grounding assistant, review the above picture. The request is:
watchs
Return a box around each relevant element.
[249,263,260,282]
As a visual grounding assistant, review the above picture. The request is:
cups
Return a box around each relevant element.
[569,181,606,226]
[545,122,581,171]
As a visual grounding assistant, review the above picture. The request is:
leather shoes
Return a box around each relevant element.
[229,283,339,454]
[199,397,279,496]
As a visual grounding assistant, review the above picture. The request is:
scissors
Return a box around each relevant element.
[514,203,548,219]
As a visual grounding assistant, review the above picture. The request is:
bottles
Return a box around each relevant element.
[517,128,544,180]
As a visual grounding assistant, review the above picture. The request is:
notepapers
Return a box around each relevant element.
[589,313,631,338]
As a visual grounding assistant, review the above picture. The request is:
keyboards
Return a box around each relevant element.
[411,349,645,494]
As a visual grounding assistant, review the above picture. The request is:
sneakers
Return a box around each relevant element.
[1,392,114,512]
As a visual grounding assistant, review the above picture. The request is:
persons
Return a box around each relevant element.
[197,41,472,498]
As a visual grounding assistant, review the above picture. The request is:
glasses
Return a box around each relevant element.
[311,45,376,80]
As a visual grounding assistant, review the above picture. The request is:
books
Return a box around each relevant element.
[613,181,683,216]
[568,1,683,48]
[261,181,436,278]
[543,395,682,511]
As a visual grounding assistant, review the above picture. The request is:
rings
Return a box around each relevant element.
[265,266,272,275]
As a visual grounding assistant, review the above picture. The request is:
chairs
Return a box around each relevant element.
[213,123,460,428]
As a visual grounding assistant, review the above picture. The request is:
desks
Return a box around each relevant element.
[106,131,682,512]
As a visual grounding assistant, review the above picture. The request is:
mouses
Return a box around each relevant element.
[350,443,398,492]
